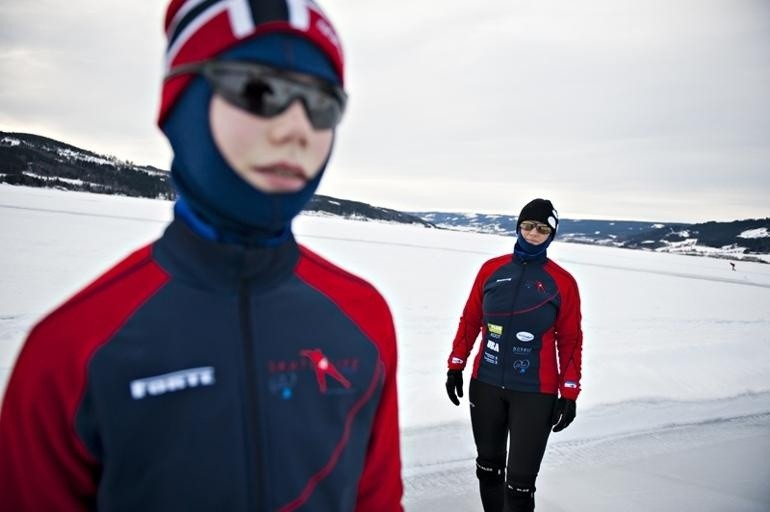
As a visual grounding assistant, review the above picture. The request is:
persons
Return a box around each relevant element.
[730,262,735,271]
[0,0,405,512]
[446,198,583,512]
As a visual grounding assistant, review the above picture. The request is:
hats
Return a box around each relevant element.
[516,198,559,234]
[158,1,345,128]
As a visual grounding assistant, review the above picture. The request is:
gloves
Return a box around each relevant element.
[446,370,463,406]
[552,397,577,432]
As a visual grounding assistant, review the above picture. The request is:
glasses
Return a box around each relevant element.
[519,220,552,234]
[163,62,349,129]
